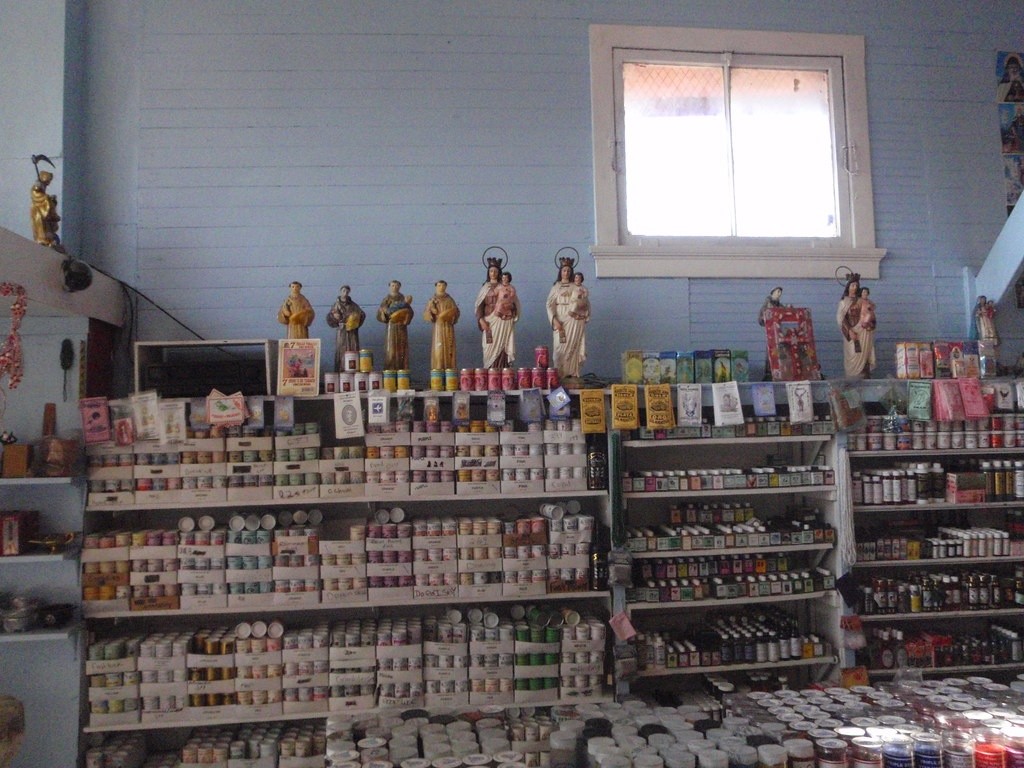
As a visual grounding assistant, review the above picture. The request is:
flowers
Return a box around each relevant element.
[1,282,28,391]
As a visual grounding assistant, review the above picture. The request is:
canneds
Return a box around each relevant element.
[75,342,1024,768]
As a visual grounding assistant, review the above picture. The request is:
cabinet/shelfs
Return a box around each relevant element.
[614,377,1024,694]
[0,226,614,768]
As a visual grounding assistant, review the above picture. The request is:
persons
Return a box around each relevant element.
[424,279,460,371]
[31,171,61,246]
[377,279,414,372]
[277,282,315,339]
[758,285,793,382]
[545,258,591,379]
[474,254,521,372]
[974,295,1001,348]
[836,273,877,379]
[326,284,367,375]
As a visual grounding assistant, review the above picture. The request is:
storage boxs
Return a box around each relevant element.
[3,443,35,478]
[896,340,996,379]
[622,350,749,386]
[1,511,26,555]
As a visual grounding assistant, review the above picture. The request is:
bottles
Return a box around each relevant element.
[622,458,1024,670]
